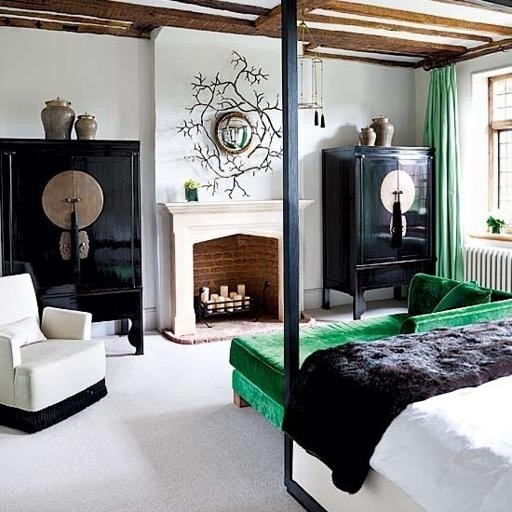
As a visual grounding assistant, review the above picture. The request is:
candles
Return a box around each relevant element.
[201,284,251,312]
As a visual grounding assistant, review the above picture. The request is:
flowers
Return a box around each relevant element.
[184,179,199,190]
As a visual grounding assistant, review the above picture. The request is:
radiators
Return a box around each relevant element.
[463,245,512,293]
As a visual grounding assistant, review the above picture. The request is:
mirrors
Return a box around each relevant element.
[215,111,254,154]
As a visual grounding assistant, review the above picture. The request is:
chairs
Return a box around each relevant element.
[0,272,107,434]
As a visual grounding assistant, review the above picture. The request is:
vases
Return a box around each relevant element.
[185,188,198,201]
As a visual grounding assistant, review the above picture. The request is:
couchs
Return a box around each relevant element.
[229,273,512,432]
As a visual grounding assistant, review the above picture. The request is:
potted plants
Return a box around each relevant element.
[486,216,506,234]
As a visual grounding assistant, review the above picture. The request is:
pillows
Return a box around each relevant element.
[432,280,493,312]
[1,316,47,348]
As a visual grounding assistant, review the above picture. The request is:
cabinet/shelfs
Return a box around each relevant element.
[0,139,143,355]
[323,146,438,319]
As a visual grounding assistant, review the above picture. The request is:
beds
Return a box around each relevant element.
[291,318,512,512]
